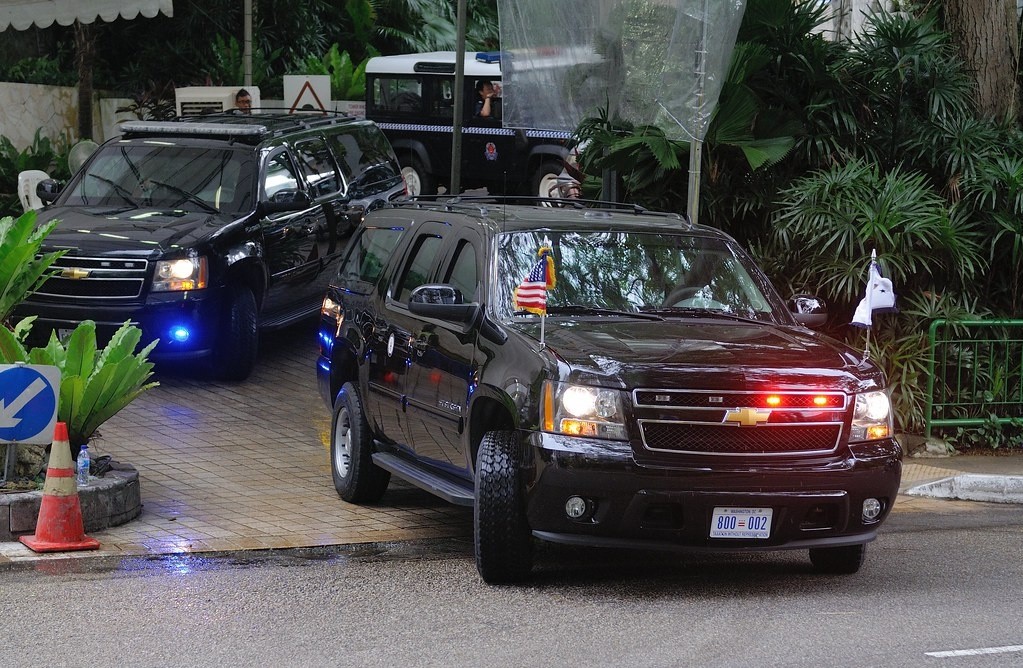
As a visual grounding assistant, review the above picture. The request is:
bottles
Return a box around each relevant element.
[77,445,90,487]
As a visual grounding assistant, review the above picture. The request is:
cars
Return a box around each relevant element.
[259,161,408,240]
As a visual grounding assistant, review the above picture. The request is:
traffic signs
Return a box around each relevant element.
[0,363,62,445]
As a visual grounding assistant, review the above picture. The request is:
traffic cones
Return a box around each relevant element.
[19,422,100,553]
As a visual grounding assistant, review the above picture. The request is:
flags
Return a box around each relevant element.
[849,262,898,331]
[512,254,557,318]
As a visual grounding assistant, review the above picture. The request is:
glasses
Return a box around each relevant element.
[238,100,252,105]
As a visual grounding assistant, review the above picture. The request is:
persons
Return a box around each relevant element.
[473,79,502,118]
[230,88,255,114]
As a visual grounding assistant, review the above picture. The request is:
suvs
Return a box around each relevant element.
[365,48,609,208]
[318,194,901,586]
[2,109,412,381]
